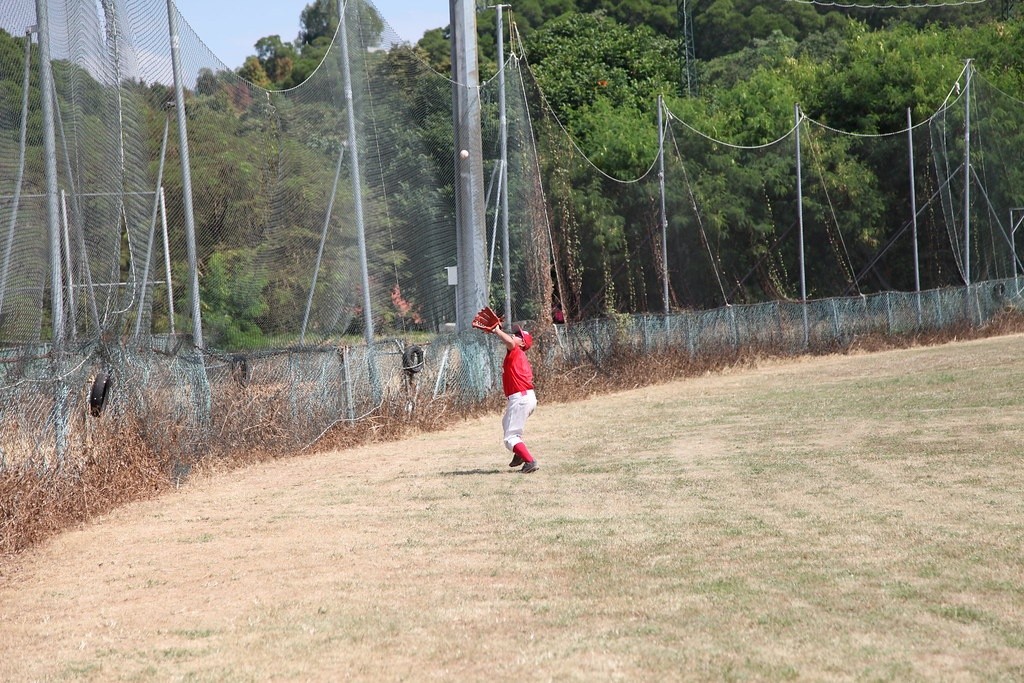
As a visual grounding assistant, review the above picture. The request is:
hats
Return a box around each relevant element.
[511,324,533,351]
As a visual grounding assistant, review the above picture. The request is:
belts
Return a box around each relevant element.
[520,390,527,396]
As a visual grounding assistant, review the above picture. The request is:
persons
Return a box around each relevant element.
[472,307,539,474]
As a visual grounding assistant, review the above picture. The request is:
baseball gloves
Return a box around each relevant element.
[470,304,502,334]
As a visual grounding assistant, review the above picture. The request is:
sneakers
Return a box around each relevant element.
[520,459,540,473]
[509,453,524,467]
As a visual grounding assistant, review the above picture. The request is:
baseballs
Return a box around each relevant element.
[459,149,470,159]
